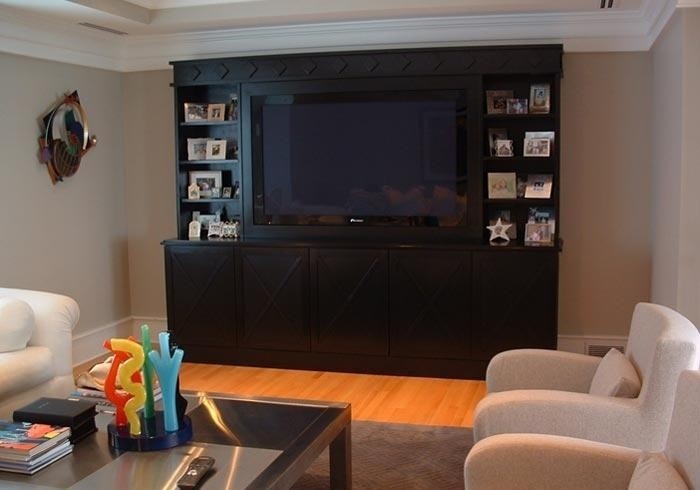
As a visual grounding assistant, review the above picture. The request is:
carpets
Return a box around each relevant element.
[287,419,475,490]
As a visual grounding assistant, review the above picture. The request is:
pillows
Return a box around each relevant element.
[0,296,34,353]
[627,449,689,490]
[587,348,642,399]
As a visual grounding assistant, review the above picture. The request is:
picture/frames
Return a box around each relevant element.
[526,82,552,115]
[485,88,516,116]
[184,103,208,122]
[188,170,222,198]
[524,173,553,199]
[505,97,529,116]
[188,182,200,199]
[221,185,232,199]
[522,138,551,157]
[187,138,214,160]
[524,223,551,243]
[206,140,226,160]
[486,218,512,241]
[488,173,517,199]
[494,139,514,157]
[522,128,557,157]
[484,124,510,157]
[189,220,201,237]
[208,103,225,121]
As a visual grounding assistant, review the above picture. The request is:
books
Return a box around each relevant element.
[12,396,101,445]
[0,419,73,474]
[67,380,163,415]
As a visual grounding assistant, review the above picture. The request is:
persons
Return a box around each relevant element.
[512,98,522,112]
[526,230,541,241]
[492,184,502,193]
[493,97,505,108]
[499,178,509,194]
[534,90,545,106]
[212,145,219,155]
[195,144,206,154]
[537,226,543,240]
[212,109,220,117]
[224,189,230,196]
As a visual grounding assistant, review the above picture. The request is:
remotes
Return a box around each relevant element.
[178,455,214,489]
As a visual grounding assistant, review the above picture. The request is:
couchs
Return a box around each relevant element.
[473,302,700,451]
[0,287,81,422]
[463,366,700,490]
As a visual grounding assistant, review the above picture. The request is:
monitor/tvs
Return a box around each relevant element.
[251,89,468,229]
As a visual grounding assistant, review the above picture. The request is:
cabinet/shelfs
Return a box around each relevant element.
[160,237,565,380]
[479,78,560,242]
[173,83,244,238]
